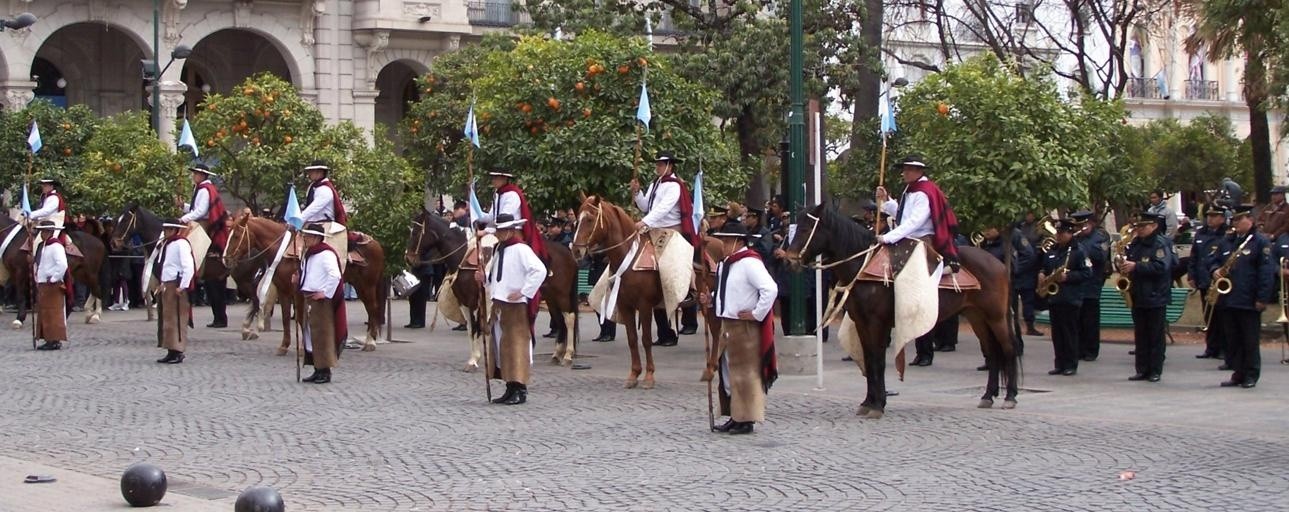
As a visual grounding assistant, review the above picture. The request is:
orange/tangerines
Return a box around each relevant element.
[205,87,292,147]
[65,124,72,130]
[937,104,949,114]
[412,77,437,133]
[514,58,647,135]
[65,149,72,154]
[482,112,488,119]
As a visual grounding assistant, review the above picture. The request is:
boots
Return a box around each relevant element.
[1025,321,1043,336]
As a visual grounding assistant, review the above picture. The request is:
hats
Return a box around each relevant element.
[490,167,517,179]
[712,221,763,238]
[707,203,730,218]
[893,154,930,169]
[37,175,59,184]
[650,151,683,164]
[36,222,64,230]
[301,223,332,238]
[189,164,217,177]
[1134,211,1159,227]
[1206,202,1254,220]
[305,160,329,172]
[162,216,189,229]
[743,205,766,216]
[495,212,527,229]
[1051,210,1093,226]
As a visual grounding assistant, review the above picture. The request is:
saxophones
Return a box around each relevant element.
[1213,235,1253,294]
[1115,229,1133,307]
[1034,249,1071,298]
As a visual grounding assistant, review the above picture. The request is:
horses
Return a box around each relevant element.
[109,201,268,330]
[402,202,582,375]
[0,211,108,331]
[567,187,727,391]
[782,199,1026,421]
[220,209,389,358]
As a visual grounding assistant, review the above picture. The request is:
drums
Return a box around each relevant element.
[393,268,421,297]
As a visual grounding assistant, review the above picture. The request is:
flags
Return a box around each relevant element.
[635,82,651,139]
[463,103,481,149]
[1155,68,1171,99]
[178,119,200,156]
[25,119,43,155]
[880,82,899,136]
[285,187,304,230]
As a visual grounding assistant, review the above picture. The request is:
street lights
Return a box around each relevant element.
[0,12,37,33]
[139,8,192,140]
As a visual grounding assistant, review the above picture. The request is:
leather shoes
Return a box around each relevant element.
[1049,367,1075,375]
[303,370,330,383]
[493,388,527,404]
[909,356,931,366]
[39,341,61,350]
[1197,351,1255,388]
[1128,373,1160,382]
[405,323,425,328]
[934,344,955,351]
[208,320,227,328]
[715,419,753,433]
[159,351,183,363]
[652,339,676,347]
[593,335,614,340]
[679,327,696,334]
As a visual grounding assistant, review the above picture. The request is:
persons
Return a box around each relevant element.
[299,159,349,384]
[174,163,229,281]
[66,213,161,311]
[149,217,196,364]
[445,153,1289,434]
[18,177,70,351]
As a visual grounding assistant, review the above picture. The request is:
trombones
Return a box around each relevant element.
[1274,257,1288,362]
[1201,281,1219,331]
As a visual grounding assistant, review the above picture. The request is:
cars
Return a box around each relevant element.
[1114,216,1197,275]
